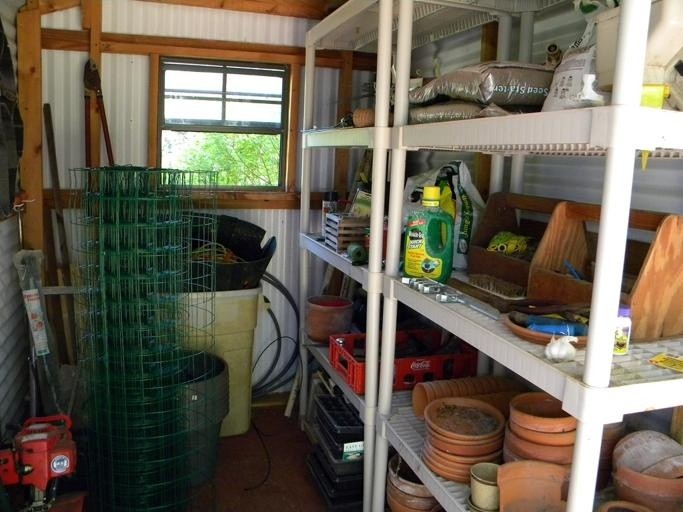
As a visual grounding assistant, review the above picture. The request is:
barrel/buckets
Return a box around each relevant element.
[179,352,231,488]
[403,186,455,283]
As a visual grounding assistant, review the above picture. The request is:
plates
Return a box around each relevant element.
[466,495,494,512]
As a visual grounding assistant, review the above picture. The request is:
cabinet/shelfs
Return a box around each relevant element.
[297,1,682,512]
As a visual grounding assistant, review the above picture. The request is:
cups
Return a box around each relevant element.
[471,461,501,510]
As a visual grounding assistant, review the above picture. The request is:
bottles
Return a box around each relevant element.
[613,306,633,357]
[321,191,338,238]
